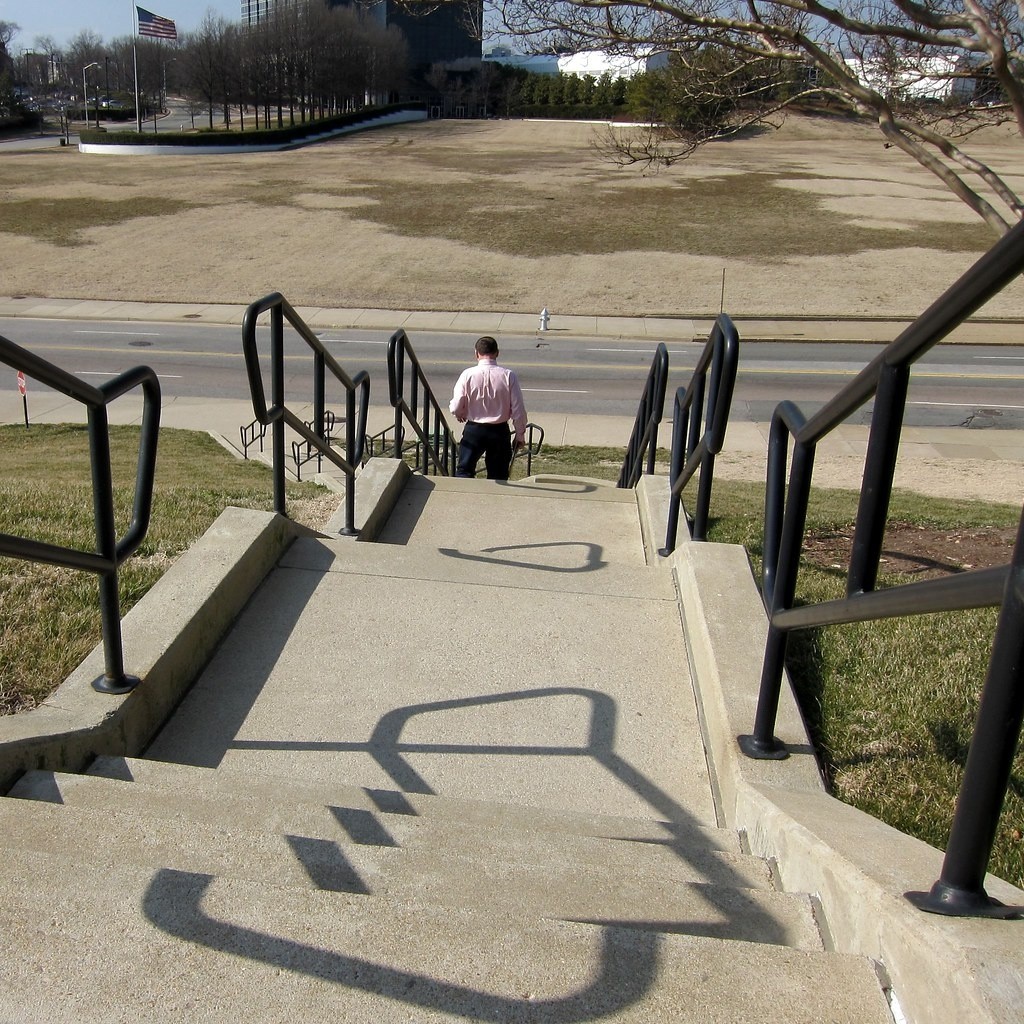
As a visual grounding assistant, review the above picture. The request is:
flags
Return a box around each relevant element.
[135,5,178,40]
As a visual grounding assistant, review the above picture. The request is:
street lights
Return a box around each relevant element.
[162,57,177,114]
[82,62,102,131]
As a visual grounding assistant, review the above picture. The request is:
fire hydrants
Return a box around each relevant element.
[538,308,552,331]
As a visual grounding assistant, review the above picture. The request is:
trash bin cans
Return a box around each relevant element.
[60,138,65,146]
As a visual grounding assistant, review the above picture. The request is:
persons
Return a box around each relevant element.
[448,335,528,480]
